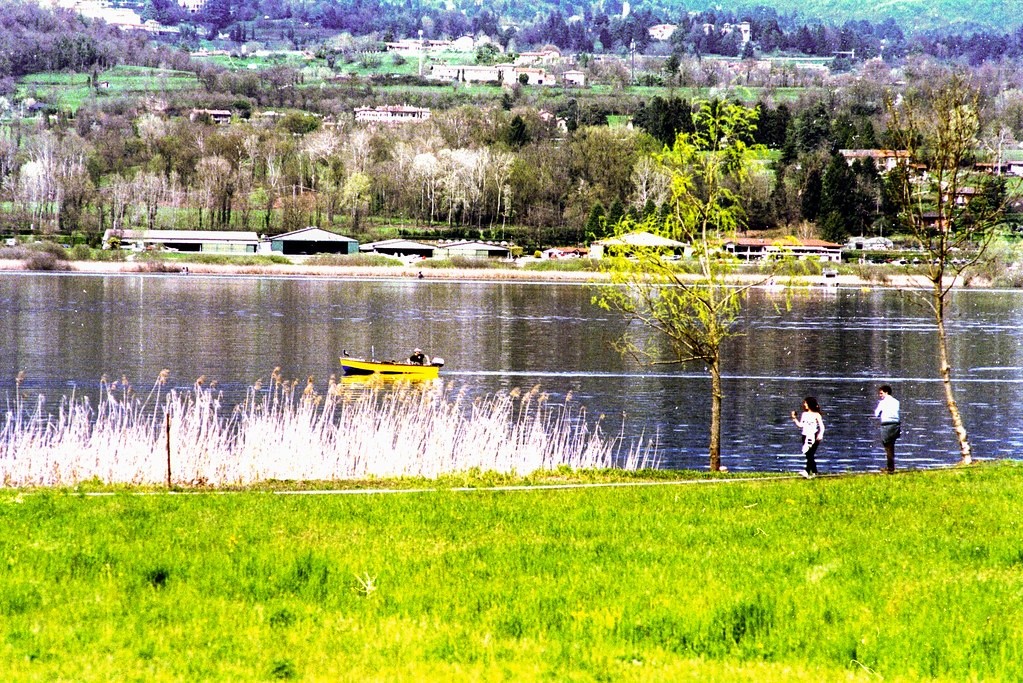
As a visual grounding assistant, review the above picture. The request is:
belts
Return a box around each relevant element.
[881,422,901,426]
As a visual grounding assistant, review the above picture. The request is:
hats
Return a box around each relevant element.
[414,348,421,352]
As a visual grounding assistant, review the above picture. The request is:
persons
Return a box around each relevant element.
[410,348,428,365]
[791,397,825,478]
[874,385,901,474]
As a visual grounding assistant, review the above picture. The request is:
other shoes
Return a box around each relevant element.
[878,468,893,475]
[809,473,818,479]
[799,470,808,479]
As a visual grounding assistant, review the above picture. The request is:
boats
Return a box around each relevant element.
[339,356,445,374]
